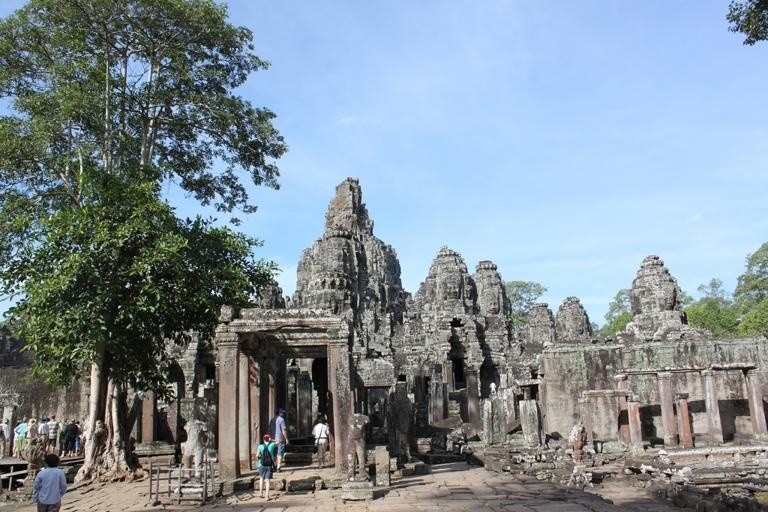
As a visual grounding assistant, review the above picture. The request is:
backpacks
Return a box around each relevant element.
[261,443,275,467]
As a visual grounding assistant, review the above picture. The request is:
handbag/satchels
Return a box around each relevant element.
[311,446,318,455]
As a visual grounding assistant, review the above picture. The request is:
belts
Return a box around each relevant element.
[314,437,329,440]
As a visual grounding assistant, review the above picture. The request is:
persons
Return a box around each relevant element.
[256,434,279,501]
[12,415,81,458]
[311,415,330,470]
[32,454,67,512]
[268,407,290,472]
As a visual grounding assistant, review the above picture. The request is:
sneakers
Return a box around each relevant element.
[259,492,270,501]
[271,467,283,473]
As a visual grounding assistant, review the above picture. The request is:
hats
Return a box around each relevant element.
[275,407,286,414]
[16,414,81,426]
[263,434,270,442]
